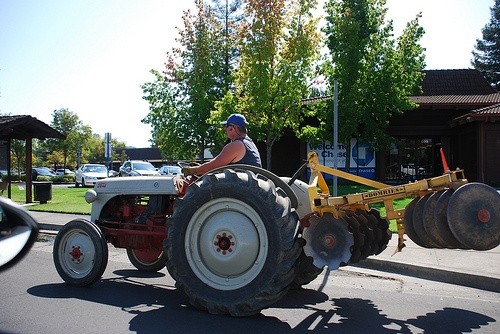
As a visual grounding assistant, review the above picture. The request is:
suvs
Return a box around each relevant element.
[118,158,162,177]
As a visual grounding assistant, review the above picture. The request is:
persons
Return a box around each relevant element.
[181,114,262,177]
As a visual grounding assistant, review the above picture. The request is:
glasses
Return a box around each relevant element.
[227,123,232,128]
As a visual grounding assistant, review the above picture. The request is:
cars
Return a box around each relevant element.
[55,169,73,176]
[74,164,109,187]
[158,165,183,176]
[32,167,55,181]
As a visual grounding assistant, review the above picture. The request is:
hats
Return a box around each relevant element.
[220,114,249,127]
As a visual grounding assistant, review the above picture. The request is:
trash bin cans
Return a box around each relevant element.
[32,182,52,204]
